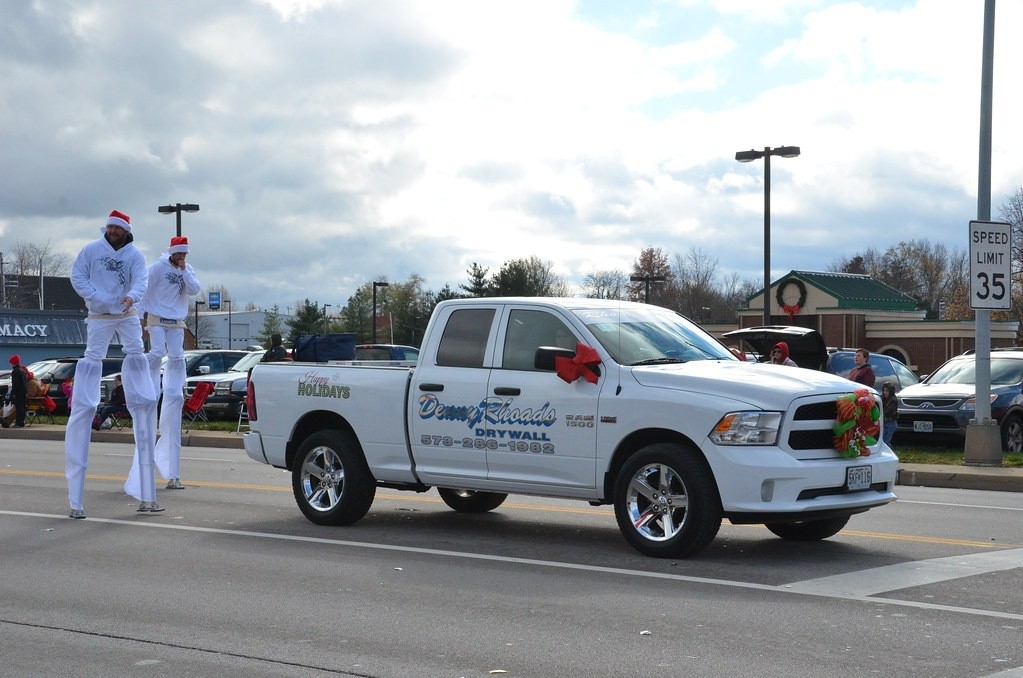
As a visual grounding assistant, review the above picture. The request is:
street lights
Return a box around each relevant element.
[372,281,389,344]
[735,145,802,329]
[195,300,205,349]
[324,303,331,335]
[223,300,231,350]
[157,203,200,238]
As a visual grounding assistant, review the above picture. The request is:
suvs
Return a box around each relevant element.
[0,345,268,422]
[722,324,921,402]
[896,348,1023,455]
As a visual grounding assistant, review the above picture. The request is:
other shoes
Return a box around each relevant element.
[92,415,104,430]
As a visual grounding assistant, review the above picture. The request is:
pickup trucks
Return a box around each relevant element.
[241,294,898,564]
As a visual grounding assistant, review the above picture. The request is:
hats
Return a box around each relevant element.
[168,237,189,254]
[100,209,131,233]
[8,354,20,365]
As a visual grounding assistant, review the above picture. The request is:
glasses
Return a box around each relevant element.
[773,350,781,353]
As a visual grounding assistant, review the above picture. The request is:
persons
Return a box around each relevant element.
[554,329,571,348]
[123,236,201,511]
[879,381,898,447]
[848,350,875,388]
[90,374,123,432]
[767,342,799,367]
[260,334,288,362]
[0,355,42,428]
[62,209,166,519]
